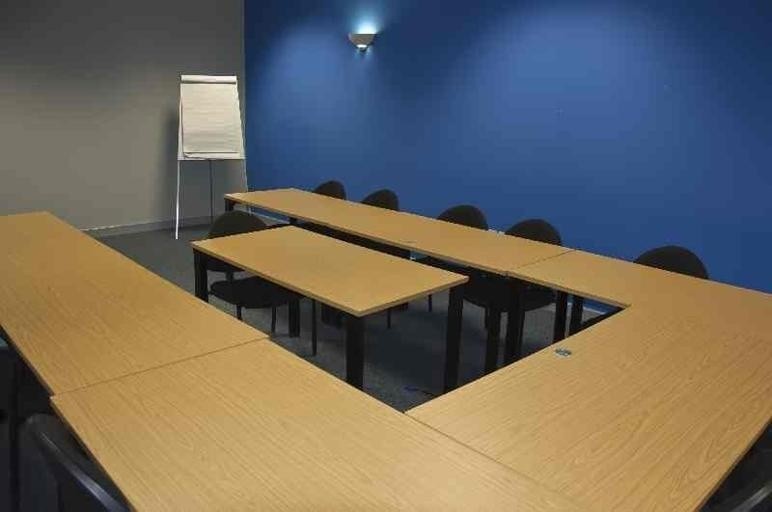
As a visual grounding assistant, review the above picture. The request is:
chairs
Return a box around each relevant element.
[343,189,399,255]
[578,244,707,329]
[740,486,769,509]
[23,408,129,509]
[198,210,319,356]
[298,180,347,240]
[454,218,564,356]
[386,205,490,330]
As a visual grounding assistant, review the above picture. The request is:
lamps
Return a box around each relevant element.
[349,33,377,53]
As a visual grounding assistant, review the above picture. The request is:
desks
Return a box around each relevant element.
[1,213,271,397]
[507,250,771,366]
[226,188,577,394]
[402,306,770,509]
[49,338,597,508]
[190,225,470,394]
